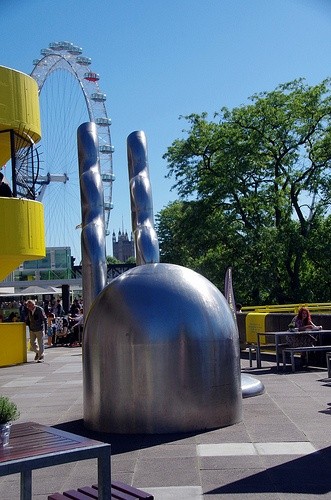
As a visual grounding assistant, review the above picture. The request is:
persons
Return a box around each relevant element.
[3,293,84,345]
[20,298,50,363]
[286,307,323,372]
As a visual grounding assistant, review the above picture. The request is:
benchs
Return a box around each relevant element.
[247,344,285,367]
[281,345,331,371]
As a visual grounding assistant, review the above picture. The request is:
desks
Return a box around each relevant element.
[257,330,331,370]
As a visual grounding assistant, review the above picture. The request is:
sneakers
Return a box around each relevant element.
[34,353,39,360]
[39,354,44,363]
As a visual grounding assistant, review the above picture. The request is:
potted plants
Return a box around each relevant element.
[288,322,295,331]
[0,395,21,449]
